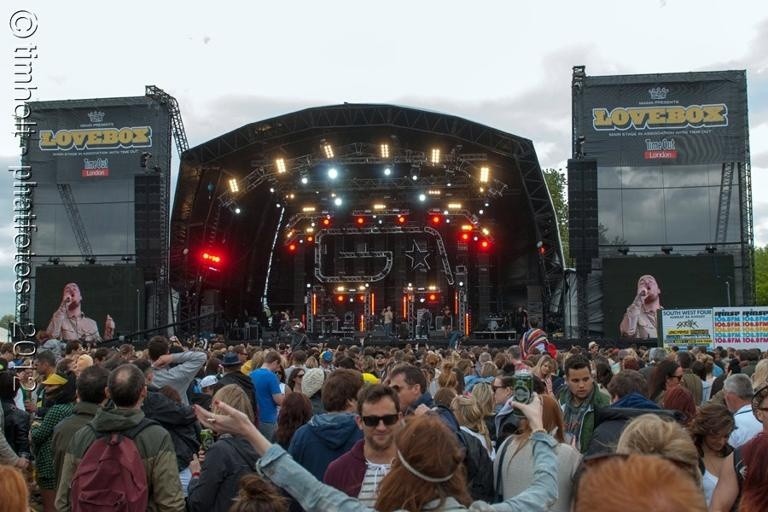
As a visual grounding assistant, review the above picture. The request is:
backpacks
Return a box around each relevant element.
[70,418,160,511]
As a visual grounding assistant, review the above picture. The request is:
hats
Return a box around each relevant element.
[301,367,325,399]
[41,372,68,386]
[200,375,219,388]
[221,352,242,365]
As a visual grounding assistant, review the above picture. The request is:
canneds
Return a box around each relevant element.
[198,428,214,452]
[512,371,535,418]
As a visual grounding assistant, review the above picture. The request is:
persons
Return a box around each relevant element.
[420,309,431,339]
[619,273,664,340]
[0,336,768,512]
[381,306,394,337]
[273,309,292,330]
[46,281,116,346]
[519,317,550,360]
[321,309,334,336]
[516,307,528,339]
[441,306,451,338]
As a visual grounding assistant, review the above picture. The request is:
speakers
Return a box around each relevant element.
[372,331,386,339]
[428,330,445,339]
[399,328,409,340]
[339,337,354,346]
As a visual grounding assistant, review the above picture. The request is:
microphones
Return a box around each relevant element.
[639,291,646,296]
[63,297,70,303]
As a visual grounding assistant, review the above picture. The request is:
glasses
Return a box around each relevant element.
[492,385,506,392]
[671,374,682,382]
[375,357,384,359]
[296,374,304,378]
[360,414,399,427]
[239,353,249,355]
[390,384,405,392]
[275,371,282,375]
